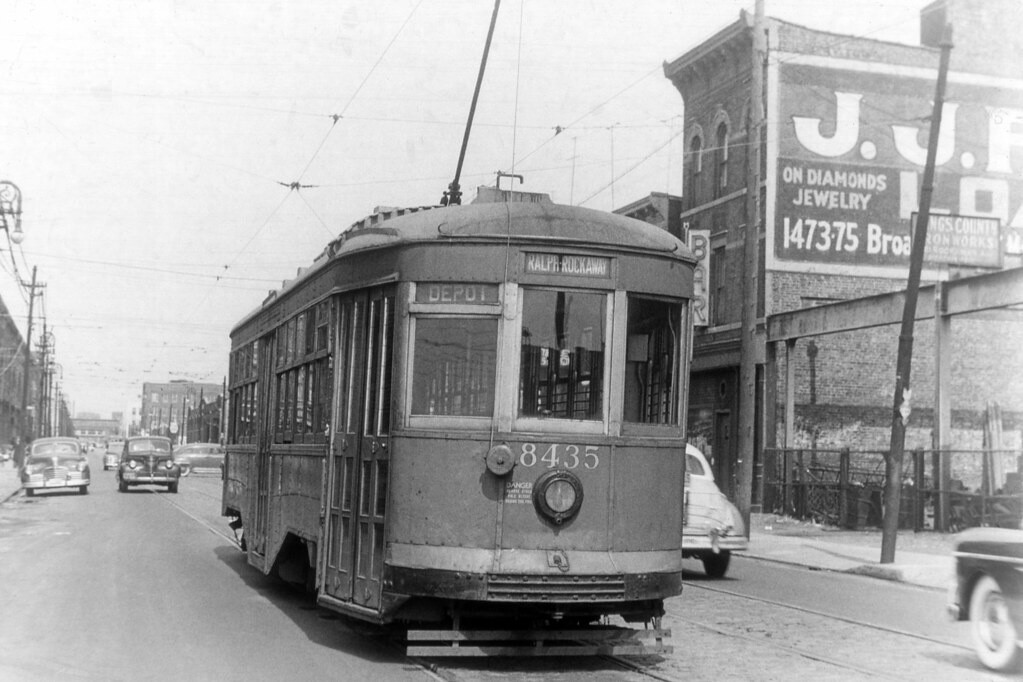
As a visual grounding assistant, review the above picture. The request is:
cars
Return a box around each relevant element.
[21,431,90,496]
[104,433,225,492]
[941,524,1023,673]
[684,442,753,589]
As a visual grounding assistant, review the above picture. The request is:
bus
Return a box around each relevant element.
[217,186,699,672]
